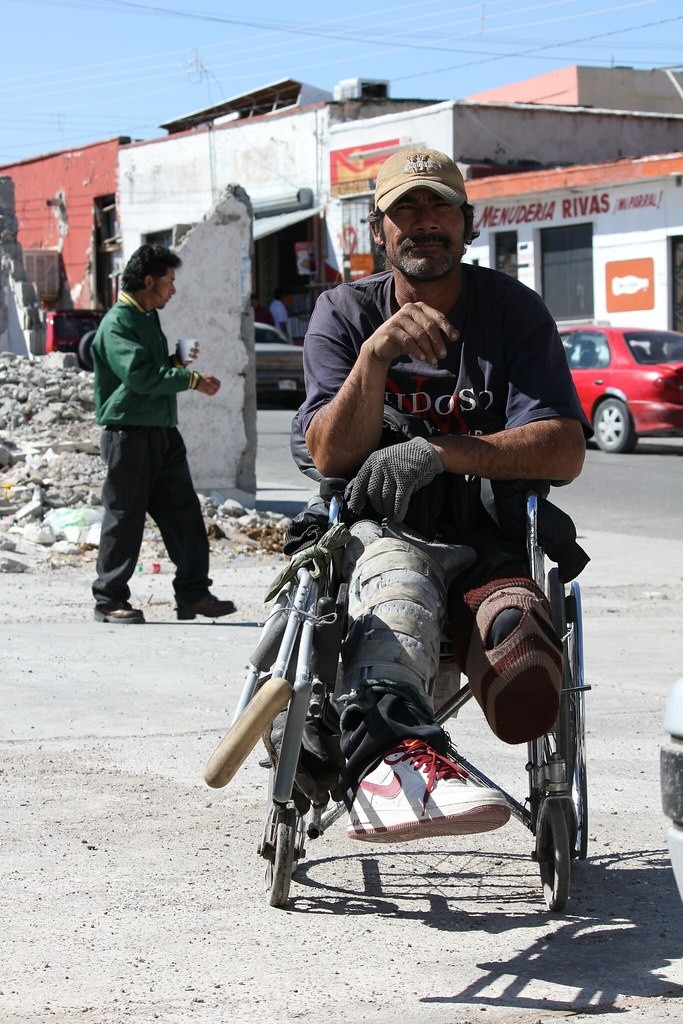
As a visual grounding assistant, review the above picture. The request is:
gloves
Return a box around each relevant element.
[343,438,445,524]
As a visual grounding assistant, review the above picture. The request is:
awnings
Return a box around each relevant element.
[252,207,317,241]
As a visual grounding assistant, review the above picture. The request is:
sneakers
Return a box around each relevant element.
[346,738,511,844]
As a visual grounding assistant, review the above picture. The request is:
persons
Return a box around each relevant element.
[290,144,596,839]
[90,244,238,623]
[251,294,275,342]
[270,289,292,340]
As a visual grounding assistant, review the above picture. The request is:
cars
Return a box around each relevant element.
[254,321,307,408]
[558,326,683,453]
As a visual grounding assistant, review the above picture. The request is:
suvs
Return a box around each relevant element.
[46,310,106,370]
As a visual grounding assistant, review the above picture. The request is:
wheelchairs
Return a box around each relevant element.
[255,477,590,913]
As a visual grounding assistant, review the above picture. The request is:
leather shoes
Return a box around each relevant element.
[176,594,237,620]
[94,602,146,623]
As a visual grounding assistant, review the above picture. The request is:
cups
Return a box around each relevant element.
[178,338,195,362]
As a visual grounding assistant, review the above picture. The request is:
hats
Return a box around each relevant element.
[374,147,468,212]
